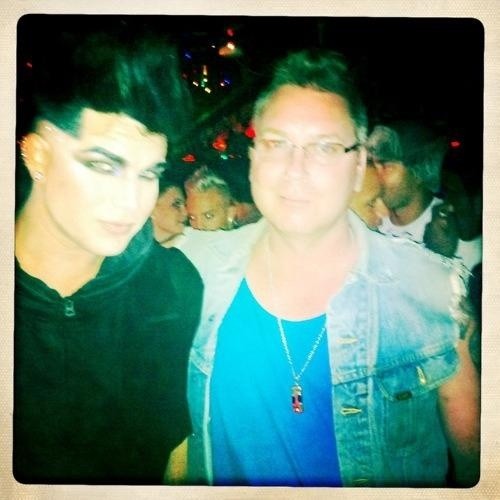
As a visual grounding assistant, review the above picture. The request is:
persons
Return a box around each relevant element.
[14,46,483,487]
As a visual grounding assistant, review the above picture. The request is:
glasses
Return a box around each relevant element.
[247,138,359,164]
